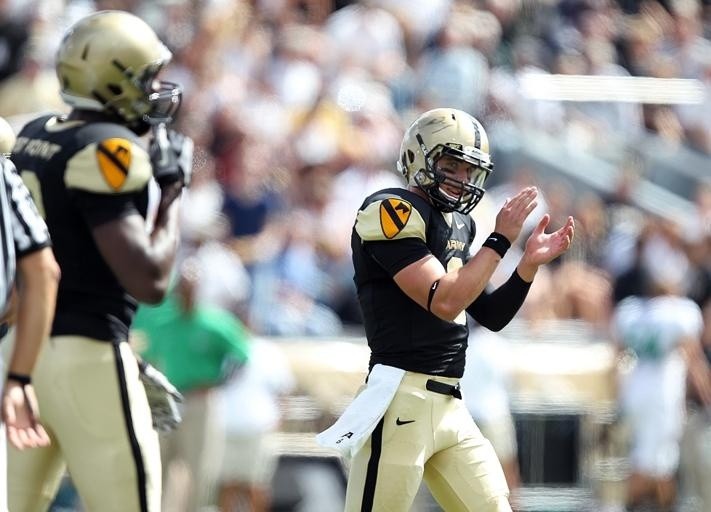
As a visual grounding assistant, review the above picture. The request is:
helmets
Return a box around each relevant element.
[55,9,183,138]
[395,107,495,216]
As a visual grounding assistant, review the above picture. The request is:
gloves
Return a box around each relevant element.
[147,127,196,191]
[137,359,187,433]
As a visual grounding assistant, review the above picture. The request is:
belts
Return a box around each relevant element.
[425,378,462,399]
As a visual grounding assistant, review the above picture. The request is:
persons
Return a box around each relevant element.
[312,107,575,511]
[2,9,194,512]
[404,1,711,511]
[1,0,104,512]
[105,1,404,511]
